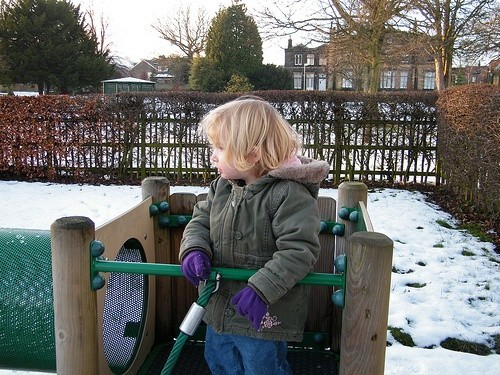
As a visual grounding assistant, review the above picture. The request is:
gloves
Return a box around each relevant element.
[181,250,212,288]
[230,286,270,331]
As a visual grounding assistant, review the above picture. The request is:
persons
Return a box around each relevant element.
[178,94,330,375]
